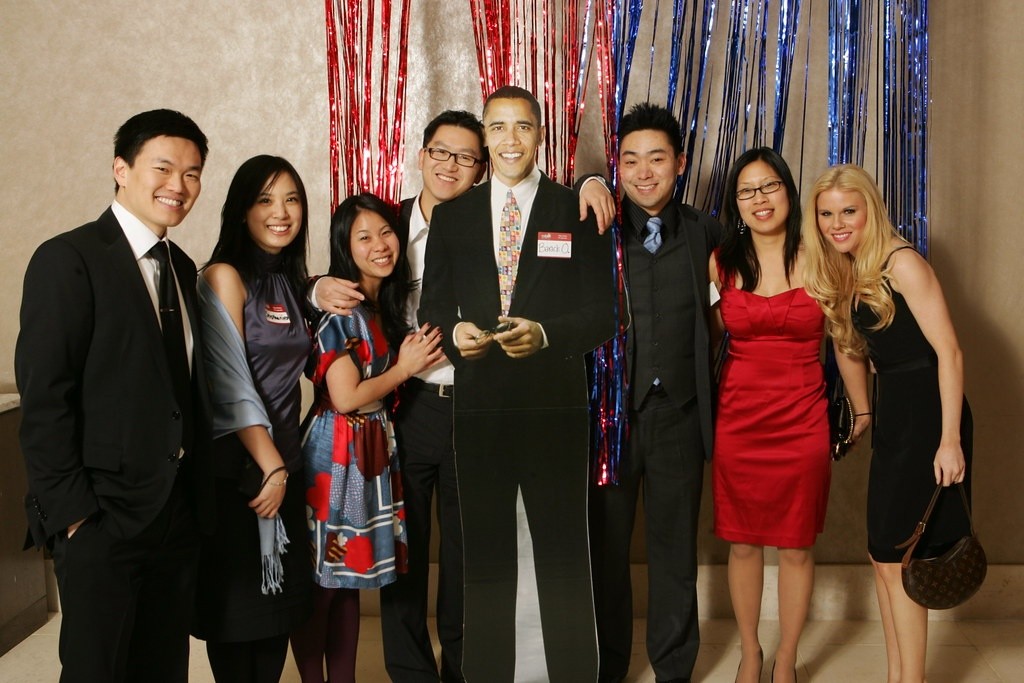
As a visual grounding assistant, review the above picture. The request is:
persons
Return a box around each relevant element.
[289,193,445,683]
[708,148,829,683]
[304,112,616,683]
[417,89,632,683]
[796,166,986,683]
[189,155,313,683]
[14,111,208,683]
[592,104,733,683]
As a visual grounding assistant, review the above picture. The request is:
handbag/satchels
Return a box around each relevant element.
[821,336,856,460]
[895,477,988,610]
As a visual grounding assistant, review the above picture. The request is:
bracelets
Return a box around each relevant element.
[855,412,873,417]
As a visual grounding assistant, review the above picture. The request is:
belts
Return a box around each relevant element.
[405,375,454,398]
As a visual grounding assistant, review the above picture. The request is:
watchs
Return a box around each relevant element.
[267,472,288,488]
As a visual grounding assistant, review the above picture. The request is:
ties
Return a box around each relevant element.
[642,216,664,254]
[147,240,195,452]
[497,189,522,317]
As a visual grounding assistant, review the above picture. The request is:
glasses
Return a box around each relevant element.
[424,146,482,167]
[735,180,784,200]
[475,320,514,347]
